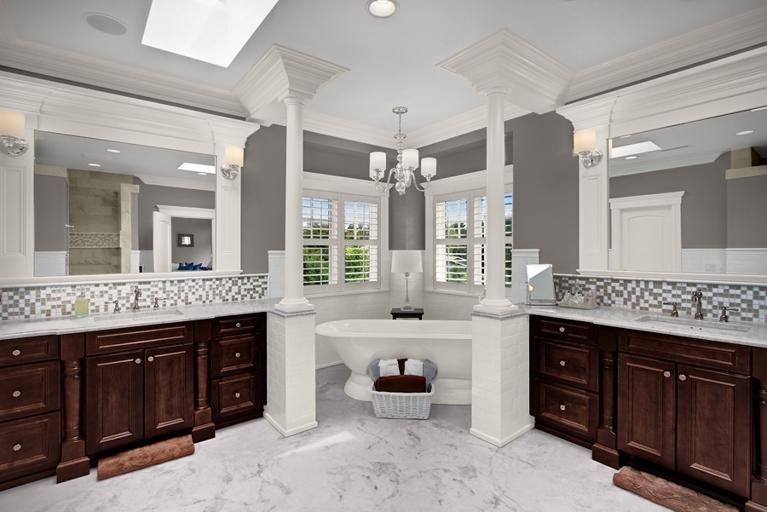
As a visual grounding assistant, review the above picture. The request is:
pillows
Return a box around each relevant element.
[177,262,192,270]
[185,262,202,270]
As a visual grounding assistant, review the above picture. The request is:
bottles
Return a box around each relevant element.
[590,286,595,306]
[583,286,588,305]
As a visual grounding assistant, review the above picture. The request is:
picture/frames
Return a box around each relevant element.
[178,234,194,248]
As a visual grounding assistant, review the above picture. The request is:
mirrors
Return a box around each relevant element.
[525,263,556,307]
[33,127,217,278]
[605,105,767,278]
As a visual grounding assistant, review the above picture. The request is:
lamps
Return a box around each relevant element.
[0,109,28,159]
[391,250,426,311]
[366,105,435,198]
[220,143,246,183]
[572,129,602,168]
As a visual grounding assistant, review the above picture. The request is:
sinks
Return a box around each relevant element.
[637,314,751,335]
[95,308,185,320]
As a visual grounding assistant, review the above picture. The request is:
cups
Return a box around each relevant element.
[74,298,89,317]
[561,289,583,306]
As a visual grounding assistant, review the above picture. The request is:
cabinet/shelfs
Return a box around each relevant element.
[210,319,268,429]
[529,313,598,450]
[82,327,196,459]
[613,334,752,512]
[0,334,62,492]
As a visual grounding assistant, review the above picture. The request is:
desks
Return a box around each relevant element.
[391,308,425,320]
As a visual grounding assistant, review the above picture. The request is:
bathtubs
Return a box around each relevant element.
[315,318,472,405]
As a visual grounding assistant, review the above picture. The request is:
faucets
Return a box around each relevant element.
[132,286,142,311]
[690,287,705,319]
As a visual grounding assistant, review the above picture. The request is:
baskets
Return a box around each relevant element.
[370,382,434,419]
